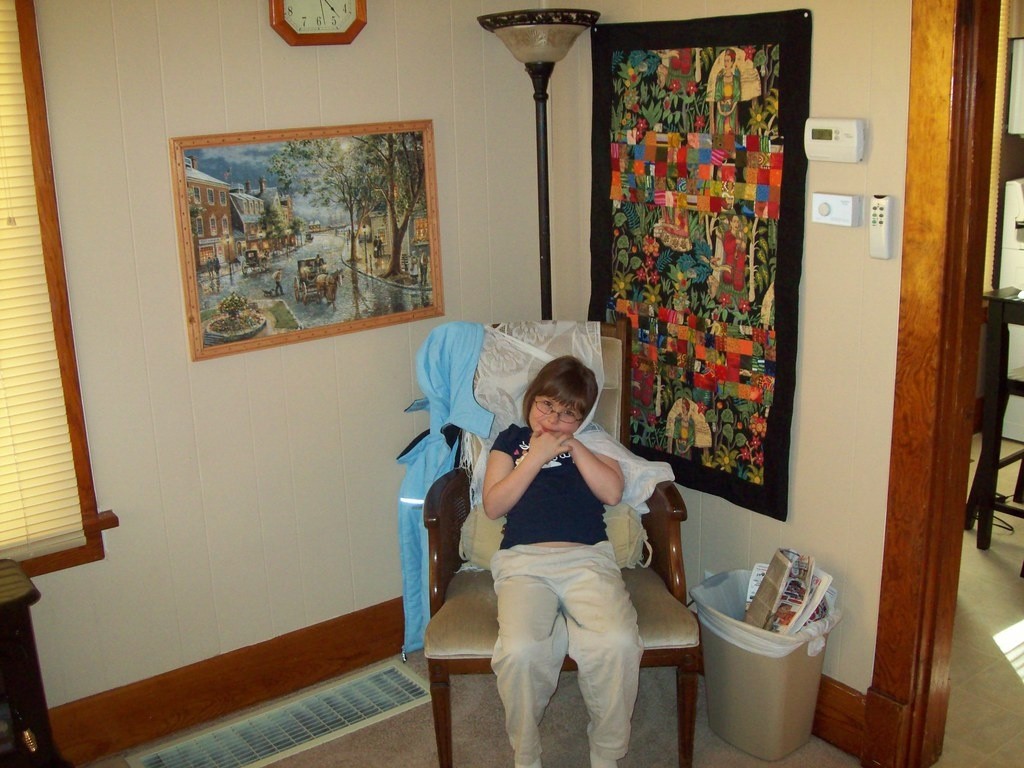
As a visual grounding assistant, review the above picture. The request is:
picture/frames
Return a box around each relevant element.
[587,7,820,522]
[169,117,444,362]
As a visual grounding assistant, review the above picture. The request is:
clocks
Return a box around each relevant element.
[268,0,367,47]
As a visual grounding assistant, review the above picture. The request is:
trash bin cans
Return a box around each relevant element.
[689,569,844,763]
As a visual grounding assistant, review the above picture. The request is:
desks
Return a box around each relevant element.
[974,288,1024,574]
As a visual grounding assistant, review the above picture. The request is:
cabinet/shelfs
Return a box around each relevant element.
[1,558,76,767]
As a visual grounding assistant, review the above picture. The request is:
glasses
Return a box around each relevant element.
[532,399,584,425]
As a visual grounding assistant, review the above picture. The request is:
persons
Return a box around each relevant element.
[482,355,645,768]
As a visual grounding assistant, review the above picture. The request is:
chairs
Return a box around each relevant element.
[417,337,708,768]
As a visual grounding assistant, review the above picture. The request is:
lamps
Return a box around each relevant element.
[475,6,604,319]
